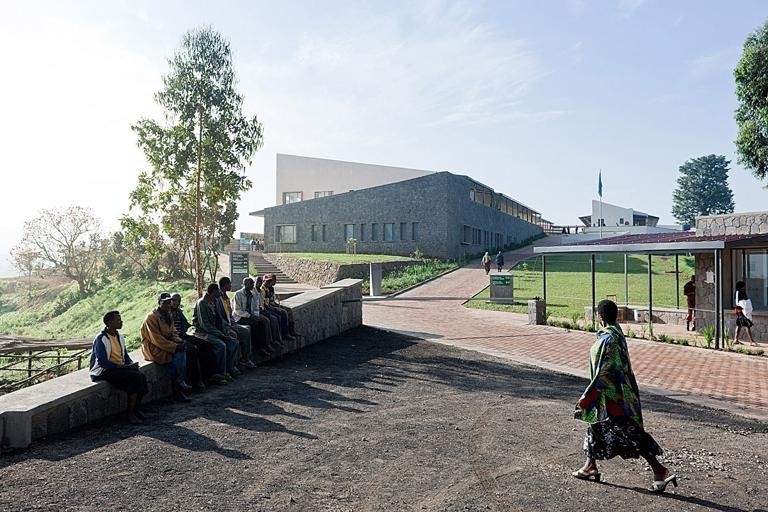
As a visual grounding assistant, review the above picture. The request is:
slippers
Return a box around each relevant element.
[647,474,678,493]
[572,467,601,482]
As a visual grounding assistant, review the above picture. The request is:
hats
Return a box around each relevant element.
[241,278,255,286]
[263,274,273,281]
[157,292,173,305]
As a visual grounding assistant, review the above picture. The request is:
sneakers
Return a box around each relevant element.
[172,360,257,402]
[255,332,302,356]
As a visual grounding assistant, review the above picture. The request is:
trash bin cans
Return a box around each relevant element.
[528,300,545,324]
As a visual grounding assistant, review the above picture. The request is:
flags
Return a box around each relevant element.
[597,171,603,198]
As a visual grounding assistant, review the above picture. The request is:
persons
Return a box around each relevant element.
[482,251,492,275]
[570,299,678,494]
[496,251,504,272]
[683,275,696,331]
[89,310,149,424]
[251,237,264,251]
[734,281,760,346]
[140,273,302,402]
